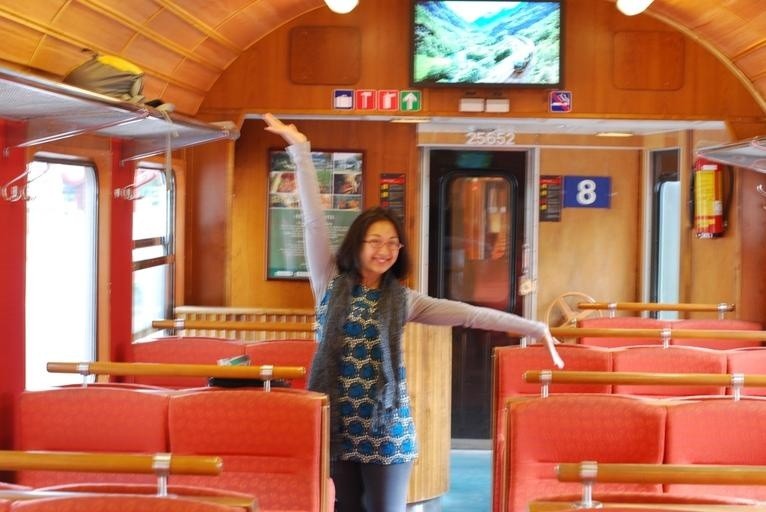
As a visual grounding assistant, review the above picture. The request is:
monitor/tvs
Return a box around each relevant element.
[410,0,562,88]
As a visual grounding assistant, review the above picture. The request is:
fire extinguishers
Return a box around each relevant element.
[690,154,735,239]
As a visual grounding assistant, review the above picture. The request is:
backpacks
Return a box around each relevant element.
[62,53,146,104]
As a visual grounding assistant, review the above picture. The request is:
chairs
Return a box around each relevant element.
[491,302,765,512]
[2,320,332,509]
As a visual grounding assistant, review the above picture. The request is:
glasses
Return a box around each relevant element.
[365,239,403,251]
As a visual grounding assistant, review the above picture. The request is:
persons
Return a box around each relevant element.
[262,112,565,512]
